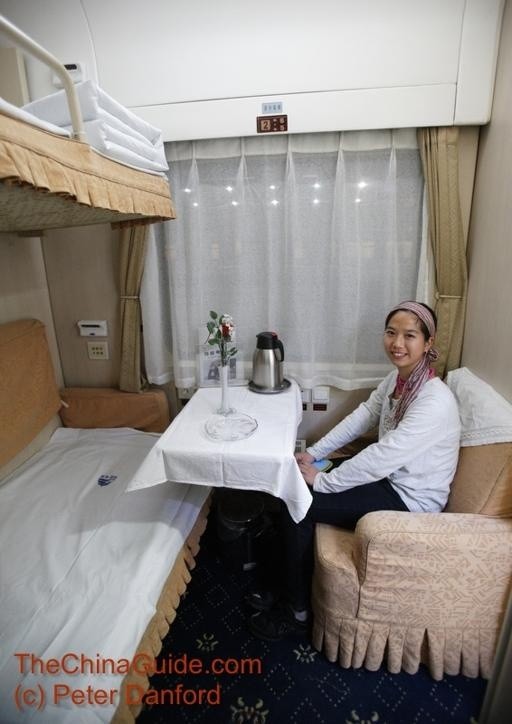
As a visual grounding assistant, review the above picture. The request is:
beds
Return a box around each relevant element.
[0,16,177,239]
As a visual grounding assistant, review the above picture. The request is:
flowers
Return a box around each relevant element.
[206,310,239,367]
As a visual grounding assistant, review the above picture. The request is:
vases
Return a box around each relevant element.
[217,366,235,414]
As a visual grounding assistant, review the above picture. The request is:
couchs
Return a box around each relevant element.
[0,320,214,724]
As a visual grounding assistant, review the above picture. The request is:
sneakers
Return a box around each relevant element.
[245,591,311,642]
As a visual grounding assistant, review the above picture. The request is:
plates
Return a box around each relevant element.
[204,413,259,442]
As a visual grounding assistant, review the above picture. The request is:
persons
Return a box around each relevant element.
[243,298,461,643]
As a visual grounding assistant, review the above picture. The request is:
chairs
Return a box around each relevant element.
[310,366,512,681]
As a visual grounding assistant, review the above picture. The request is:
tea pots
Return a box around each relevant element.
[248,331,292,386]
